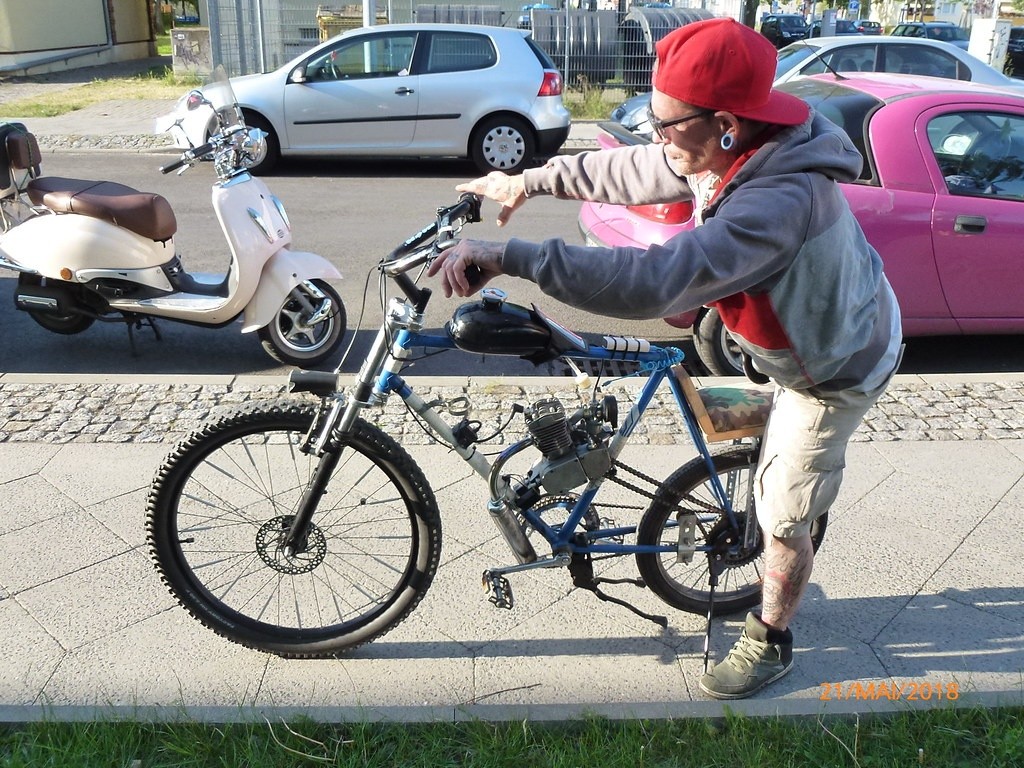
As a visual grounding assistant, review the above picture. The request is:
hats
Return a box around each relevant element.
[651,19,809,125]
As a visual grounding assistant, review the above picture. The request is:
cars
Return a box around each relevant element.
[760,14,883,49]
[173,23,572,176]
[1007,26,1024,64]
[578,71,1024,378]
[611,34,1024,141]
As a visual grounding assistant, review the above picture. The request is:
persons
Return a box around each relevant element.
[427,20,906,698]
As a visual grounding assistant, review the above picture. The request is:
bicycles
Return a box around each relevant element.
[143,190,827,676]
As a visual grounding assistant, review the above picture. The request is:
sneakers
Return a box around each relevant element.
[698,611,794,699]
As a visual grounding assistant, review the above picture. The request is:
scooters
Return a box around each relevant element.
[0,65,347,366]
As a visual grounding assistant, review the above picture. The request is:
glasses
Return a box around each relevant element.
[647,103,743,140]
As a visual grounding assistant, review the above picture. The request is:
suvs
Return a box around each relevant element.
[891,21,970,50]
